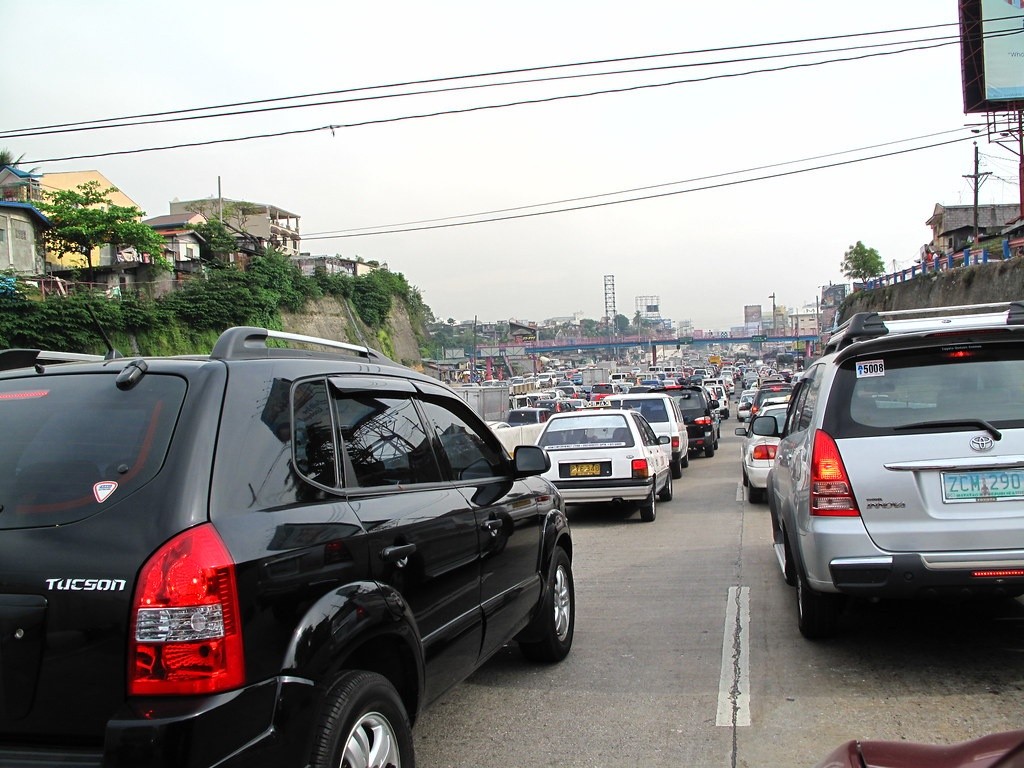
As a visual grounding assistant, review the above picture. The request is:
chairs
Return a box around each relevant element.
[612,428,632,441]
[567,429,588,444]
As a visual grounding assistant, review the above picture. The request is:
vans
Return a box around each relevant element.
[600,393,690,480]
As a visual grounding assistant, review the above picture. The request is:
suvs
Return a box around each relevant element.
[649,385,722,459]
[0,325,574,768]
[764,298,1024,644]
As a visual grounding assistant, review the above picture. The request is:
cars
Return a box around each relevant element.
[456,348,810,428]
[533,409,673,522]
[735,401,796,503]
[707,385,731,419]
